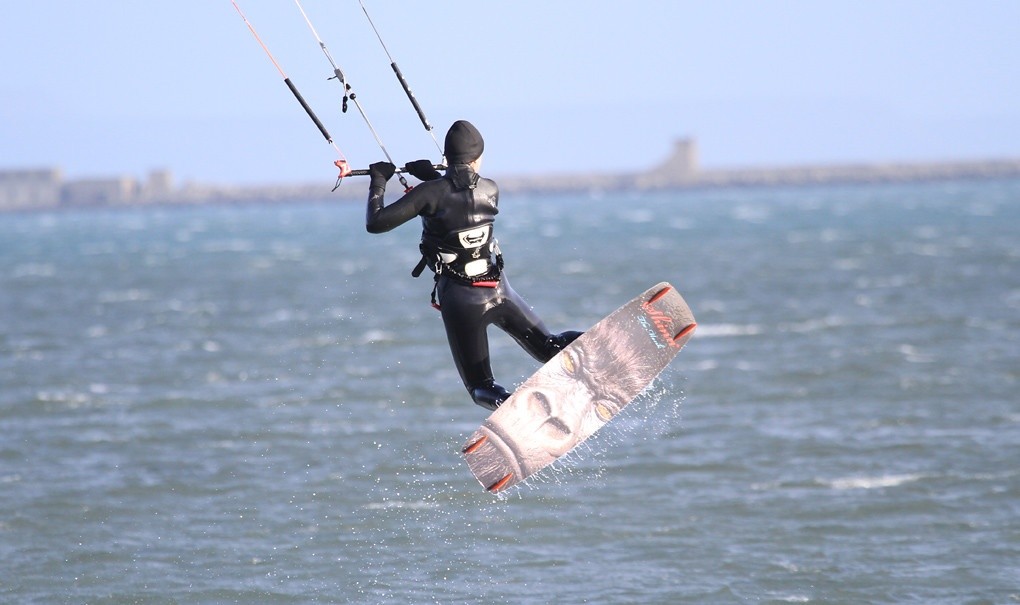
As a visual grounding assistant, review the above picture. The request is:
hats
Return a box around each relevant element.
[443,119,484,165]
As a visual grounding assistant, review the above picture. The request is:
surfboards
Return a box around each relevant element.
[461,281,698,494]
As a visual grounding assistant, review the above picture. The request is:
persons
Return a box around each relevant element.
[365,120,585,411]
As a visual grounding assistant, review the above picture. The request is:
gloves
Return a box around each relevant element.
[405,159,442,181]
[369,160,396,192]
